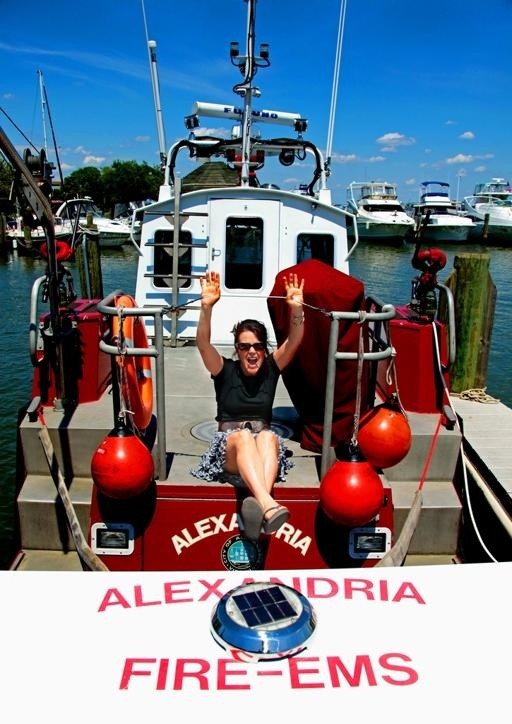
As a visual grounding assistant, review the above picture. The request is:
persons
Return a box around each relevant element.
[189,272,305,543]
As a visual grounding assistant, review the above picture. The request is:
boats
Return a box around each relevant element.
[347,178,511,243]
[3,196,142,246]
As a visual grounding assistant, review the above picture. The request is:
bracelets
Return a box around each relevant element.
[290,311,304,318]
[288,317,305,328]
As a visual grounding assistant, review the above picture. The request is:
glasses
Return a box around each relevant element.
[234,341,269,352]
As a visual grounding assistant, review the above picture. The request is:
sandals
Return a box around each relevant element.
[262,504,291,535]
[239,495,265,543]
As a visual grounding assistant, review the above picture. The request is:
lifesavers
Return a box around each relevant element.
[39,241,72,262]
[112,294,153,429]
